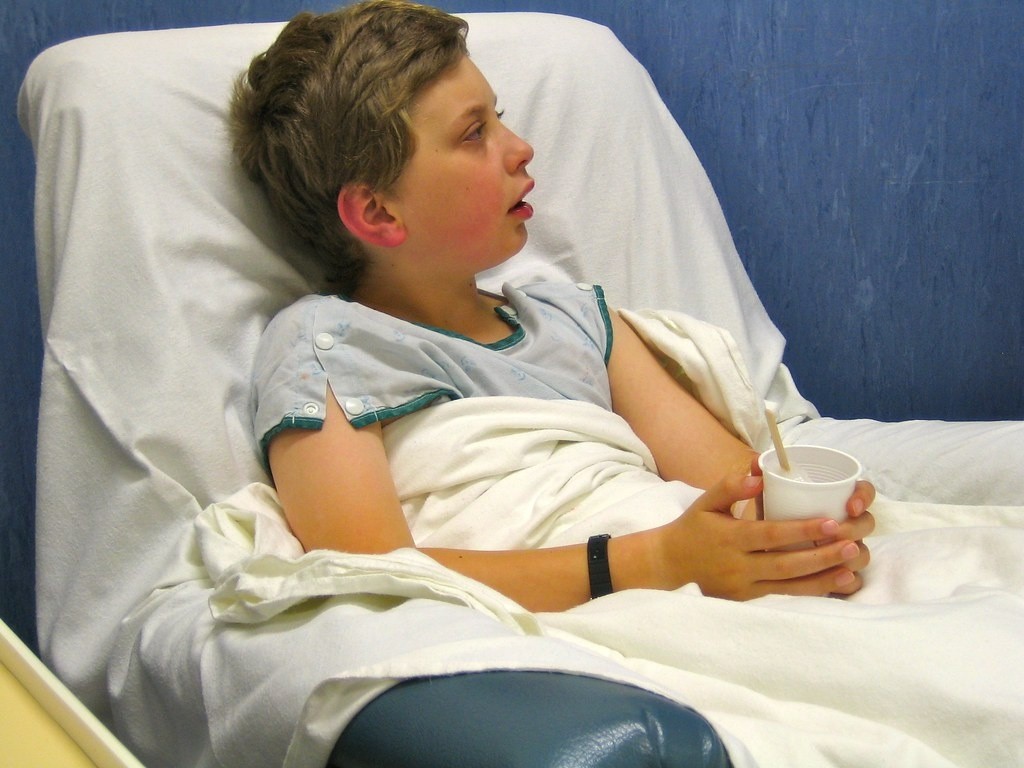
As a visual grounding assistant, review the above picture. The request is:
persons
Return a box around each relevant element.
[229,0,879,614]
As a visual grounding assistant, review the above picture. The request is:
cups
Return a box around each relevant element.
[756,444,863,598]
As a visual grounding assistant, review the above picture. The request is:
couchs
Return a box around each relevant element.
[17,16,1024,768]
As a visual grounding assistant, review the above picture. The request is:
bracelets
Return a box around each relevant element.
[584,532,617,598]
